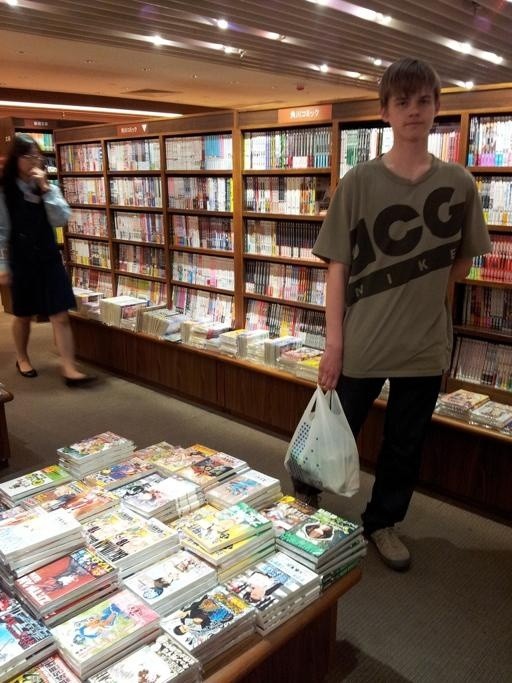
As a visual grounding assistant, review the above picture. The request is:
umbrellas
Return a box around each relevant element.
[16,357,38,378]
[63,374,98,388]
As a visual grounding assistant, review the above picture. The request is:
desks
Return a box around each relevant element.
[184,563,363,683]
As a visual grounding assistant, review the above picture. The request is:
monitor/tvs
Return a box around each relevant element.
[426,110,512,437]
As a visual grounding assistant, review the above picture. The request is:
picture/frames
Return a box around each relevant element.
[184,563,363,683]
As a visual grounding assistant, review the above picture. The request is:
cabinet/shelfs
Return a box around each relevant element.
[0,82,512,532]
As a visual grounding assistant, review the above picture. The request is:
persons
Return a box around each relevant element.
[289,57,491,572]
[0,132,97,388]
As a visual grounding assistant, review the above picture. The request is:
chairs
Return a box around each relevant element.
[296,493,321,509]
[370,527,411,572]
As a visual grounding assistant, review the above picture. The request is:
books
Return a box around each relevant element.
[339,128,394,178]
[426,110,512,437]
[58,143,113,298]
[69,286,324,383]
[105,138,168,305]
[162,134,235,324]
[0,431,369,683]
[243,127,332,350]
